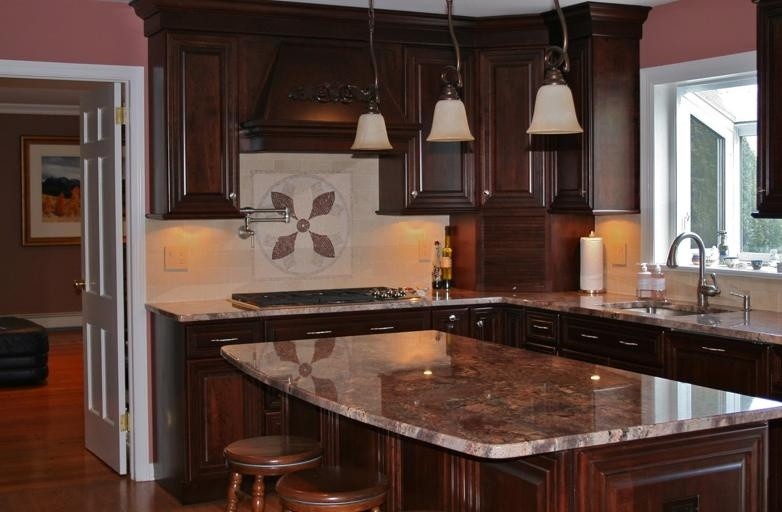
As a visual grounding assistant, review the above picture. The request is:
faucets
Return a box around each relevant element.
[666,232,722,314]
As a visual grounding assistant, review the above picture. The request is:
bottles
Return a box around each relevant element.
[442,233,452,290]
[431,240,442,290]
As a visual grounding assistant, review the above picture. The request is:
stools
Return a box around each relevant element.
[222,436,324,512]
[276,464,389,512]
[0,312,49,386]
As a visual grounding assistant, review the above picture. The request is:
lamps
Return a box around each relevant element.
[426,0,475,143]
[350,1,395,152]
[527,1,584,135]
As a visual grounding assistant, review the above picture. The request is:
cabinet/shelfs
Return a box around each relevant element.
[392,441,559,512]
[752,0,782,219]
[433,308,502,341]
[164,32,241,215]
[504,309,557,354]
[403,47,479,210]
[281,393,392,487]
[267,320,429,434]
[476,45,547,207]
[548,42,595,210]
[186,323,266,481]
[558,313,660,377]
[661,330,769,402]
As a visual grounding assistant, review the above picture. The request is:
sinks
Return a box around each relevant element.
[596,300,737,318]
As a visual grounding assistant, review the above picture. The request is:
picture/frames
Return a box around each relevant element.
[20,134,84,248]
